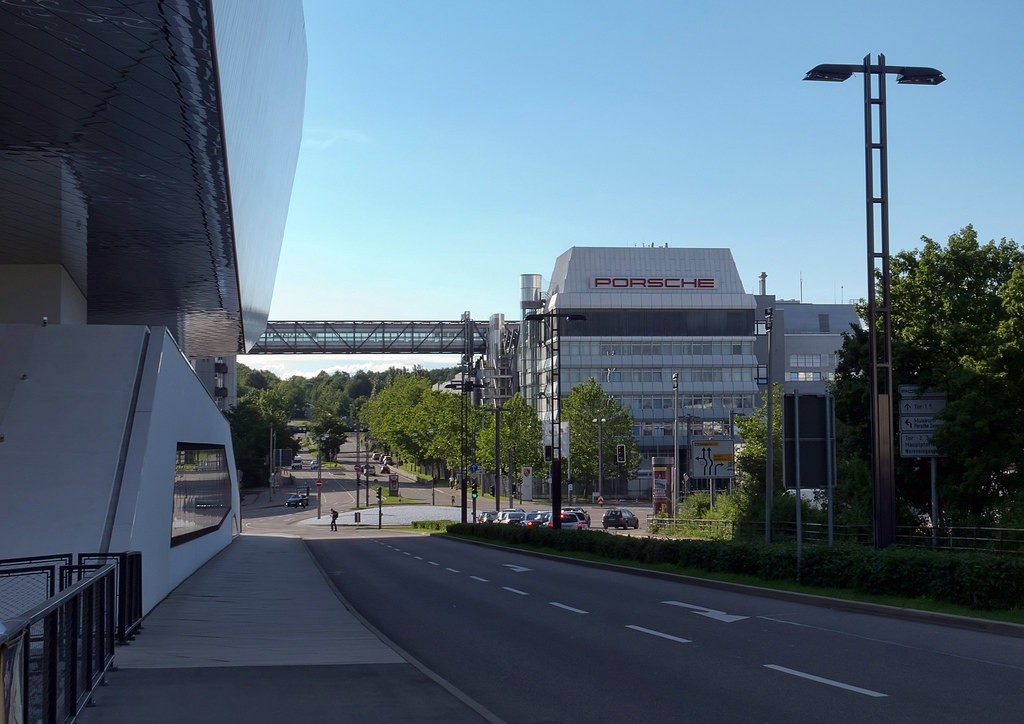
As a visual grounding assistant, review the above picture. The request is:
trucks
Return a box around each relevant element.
[291,456,303,469]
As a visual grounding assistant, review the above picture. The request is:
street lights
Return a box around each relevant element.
[481,406,511,512]
[524,313,588,529]
[272,431,277,494]
[672,373,679,529]
[763,307,774,542]
[445,379,486,524]
[802,53,946,550]
[268,421,273,502]
[591,417,607,496]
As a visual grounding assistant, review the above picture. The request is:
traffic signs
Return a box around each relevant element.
[899,417,945,431]
[899,397,947,415]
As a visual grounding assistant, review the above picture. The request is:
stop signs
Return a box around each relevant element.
[354,465,360,470]
[315,480,323,487]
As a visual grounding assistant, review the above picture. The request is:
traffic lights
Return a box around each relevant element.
[375,487,381,498]
[489,485,496,497]
[519,478,523,484]
[471,483,478,499]
[616,444,627,463]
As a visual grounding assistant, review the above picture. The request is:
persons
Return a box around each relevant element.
[330,508,338,532]
[434,473,459,489]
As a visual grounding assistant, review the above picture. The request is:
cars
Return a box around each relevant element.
[372,453,380,460]
[384,459,393,466]
[383,456,392,462]
[285,494,308,508]
[380,466,390,474]
[602,509,639,530]
[360,464,366,472]
[379,454,388,462]
[363,465,375,476]
[476,507,591,530]
[310,460,319,470]
